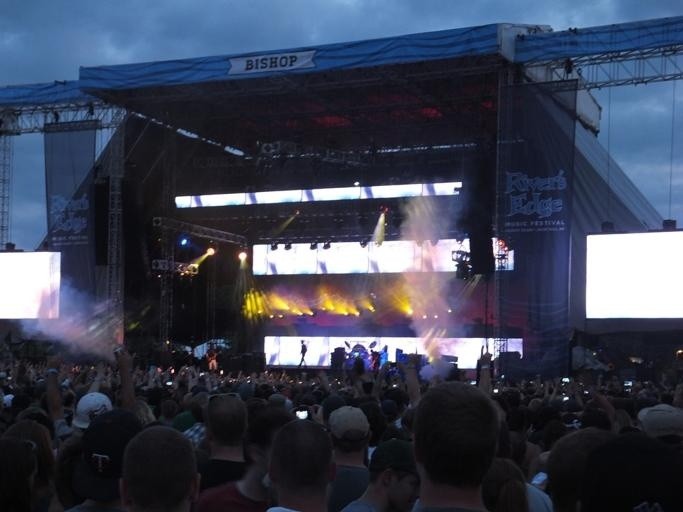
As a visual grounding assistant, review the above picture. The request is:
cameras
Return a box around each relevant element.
[165,381,172,386]
[561,377,570,383]
[113,347,125,359]
[624,380,633,387]
[398,354,408,364]
[294,406,313,422]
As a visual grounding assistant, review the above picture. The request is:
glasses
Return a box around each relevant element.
[208,392,240,402]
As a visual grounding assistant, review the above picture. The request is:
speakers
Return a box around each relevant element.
[461,120,497,274]
[121,176,154,280]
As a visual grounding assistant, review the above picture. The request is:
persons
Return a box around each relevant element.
[2,338,682,512]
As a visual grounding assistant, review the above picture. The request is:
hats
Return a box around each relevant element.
[74,408,142,502]
[330,405,370,440]
[637,404,683,448]
[71,393,112,430]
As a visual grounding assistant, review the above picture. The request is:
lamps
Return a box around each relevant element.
[206,243,219,256]
[232,247,249,262]
